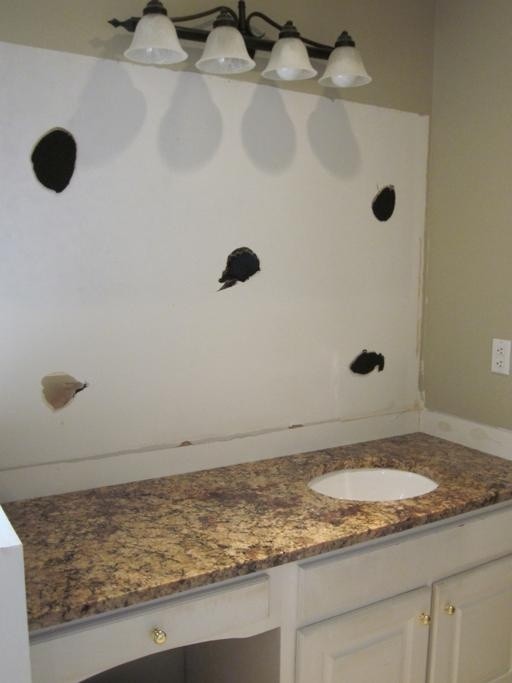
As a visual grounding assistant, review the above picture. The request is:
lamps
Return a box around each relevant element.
[99,2,372,91]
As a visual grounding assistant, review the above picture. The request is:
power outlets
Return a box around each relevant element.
[490,337,512,376]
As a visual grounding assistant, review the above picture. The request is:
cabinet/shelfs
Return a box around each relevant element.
[31,573,273,683]
[294,550,512,681]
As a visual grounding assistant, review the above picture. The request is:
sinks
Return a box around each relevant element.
[304,458,441,501]
